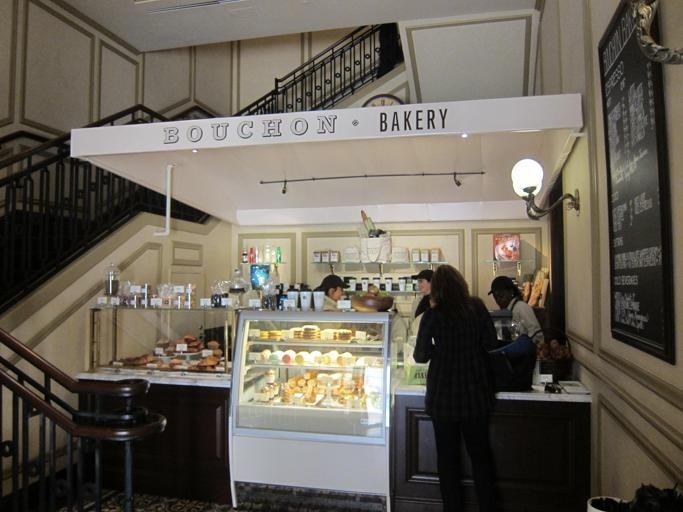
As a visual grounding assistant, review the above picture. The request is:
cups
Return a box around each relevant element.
[348,277,357,291]
[361,277,369,292]
[372,277,380,289]
[385,277,392,292]
[398,277,406,291]
[313,289,325,312]
[299,289,313,311]
[286,288,299,311]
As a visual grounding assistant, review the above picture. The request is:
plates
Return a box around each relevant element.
[299,392,325,406]
[272,392,302,405]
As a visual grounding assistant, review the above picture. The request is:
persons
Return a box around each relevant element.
[412,262,506,511]
[308,273,351,331]
[487,275,547,347]
[409,268,434,320]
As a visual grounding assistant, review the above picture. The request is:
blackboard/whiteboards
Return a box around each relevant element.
[597,0,676,366]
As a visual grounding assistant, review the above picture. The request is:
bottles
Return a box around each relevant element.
[104,262,119,297]
[361,211,376,233]
[230,269,245,294]
[240,245,282,263]
[211,282,229,308]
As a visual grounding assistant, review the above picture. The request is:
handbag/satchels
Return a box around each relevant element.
[483,343,508,376]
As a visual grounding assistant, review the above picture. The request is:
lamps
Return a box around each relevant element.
[509,157,580,223]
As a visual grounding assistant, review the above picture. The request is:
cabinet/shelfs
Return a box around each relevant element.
[310,261,448,295]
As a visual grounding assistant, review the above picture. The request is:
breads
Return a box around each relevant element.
[108,335,223,372]
[280,370,366,408]
[248,324,352,342]
[246,349,352,367]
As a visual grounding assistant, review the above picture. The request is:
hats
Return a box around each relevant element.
[488,276,511,294]
[411,270,433,278]
[320,274,350,289]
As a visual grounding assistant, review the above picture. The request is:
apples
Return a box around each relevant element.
[354,285,393,297]
